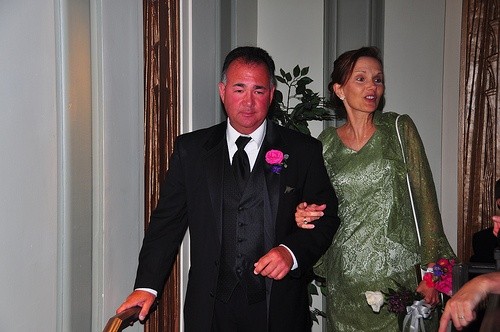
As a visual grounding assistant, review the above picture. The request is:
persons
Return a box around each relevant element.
[116,46,340,332]
[294,46,455,332]
[439,178,500,332]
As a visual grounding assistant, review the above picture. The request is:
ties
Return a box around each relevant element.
[231,137,251,192]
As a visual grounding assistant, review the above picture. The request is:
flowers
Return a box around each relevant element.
[364,276,444,316]
[265,149,290,175]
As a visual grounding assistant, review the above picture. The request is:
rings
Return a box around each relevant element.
[304,216,307,224]
[458,316,465,320]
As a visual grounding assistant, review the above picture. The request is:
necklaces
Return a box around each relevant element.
[345,122,374,150]
